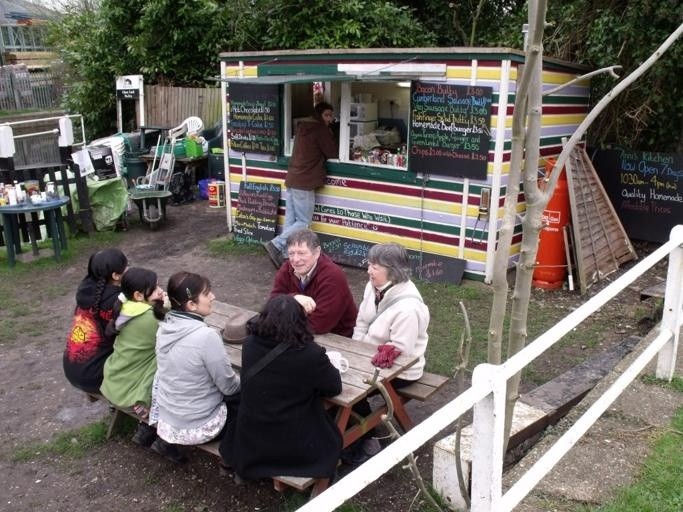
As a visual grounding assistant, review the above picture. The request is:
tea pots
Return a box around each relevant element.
[29,195,43,206]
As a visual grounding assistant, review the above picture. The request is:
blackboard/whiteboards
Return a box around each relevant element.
[311,230,468,287]
[408,81,493,181]
[229,81,283,157]
[232,181,282,244]
[615,147,683,243]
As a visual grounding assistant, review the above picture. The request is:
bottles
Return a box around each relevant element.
[395,145,405,155]
[0,182,23,207]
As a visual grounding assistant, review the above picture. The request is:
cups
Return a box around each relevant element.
[323,351,349,374]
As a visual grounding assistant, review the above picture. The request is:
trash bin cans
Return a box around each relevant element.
[124,149,149,188]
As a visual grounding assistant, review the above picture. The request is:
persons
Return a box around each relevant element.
[270,227,358,339]
[147,269,242,467]
[219,291,345,488]
[341,240,432,469]
[259,101,340,273]
[97,265,171,449]
[61,247,130,404]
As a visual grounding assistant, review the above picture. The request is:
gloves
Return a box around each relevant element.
[371,345,401,369]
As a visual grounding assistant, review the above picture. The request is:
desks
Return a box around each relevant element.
[156,292,421,504]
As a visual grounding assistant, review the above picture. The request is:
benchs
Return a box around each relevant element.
[389,367,450,435]
[87,392,343,502]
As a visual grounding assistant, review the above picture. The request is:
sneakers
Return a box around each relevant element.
[150,438,188,465]
[265,241,283,270]
[346,437,381,464]
[131,422,156,454]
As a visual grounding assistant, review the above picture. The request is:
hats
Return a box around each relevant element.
[221,311,260,343]
